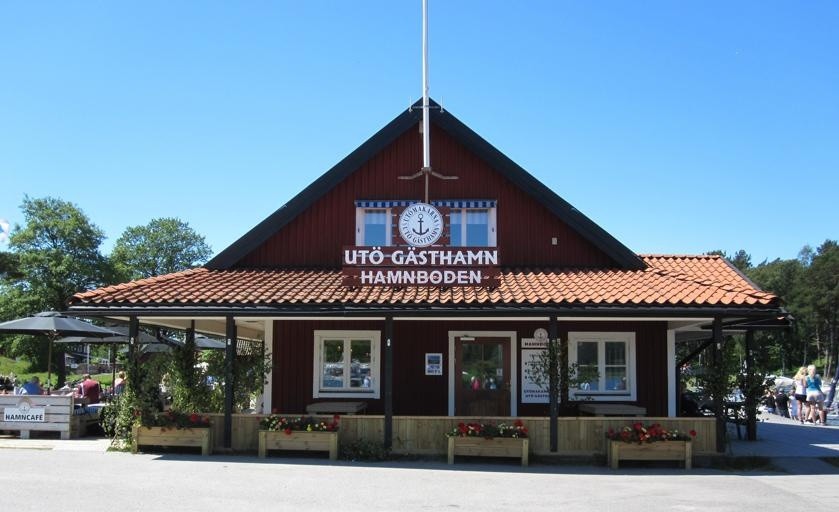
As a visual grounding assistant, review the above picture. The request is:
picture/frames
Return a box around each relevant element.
[425,353,443,377]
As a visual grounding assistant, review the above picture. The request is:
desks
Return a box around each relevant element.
[307,402,368,415]
[578,404,648,417]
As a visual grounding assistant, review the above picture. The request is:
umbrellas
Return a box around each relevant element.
[115,343,175,353]
[194,336,226,349]
[52,327,161,396]
[0,310,126,395]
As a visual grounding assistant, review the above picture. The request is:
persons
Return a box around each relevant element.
[114,372,126,394]
[766,364,827,425]
[77,374,100,403]
[11,375,43,395]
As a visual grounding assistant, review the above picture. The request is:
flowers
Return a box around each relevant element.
[256,407,341,434]
[133,408,214,433]
[445,419,530,441]
[608,422,697,446]
